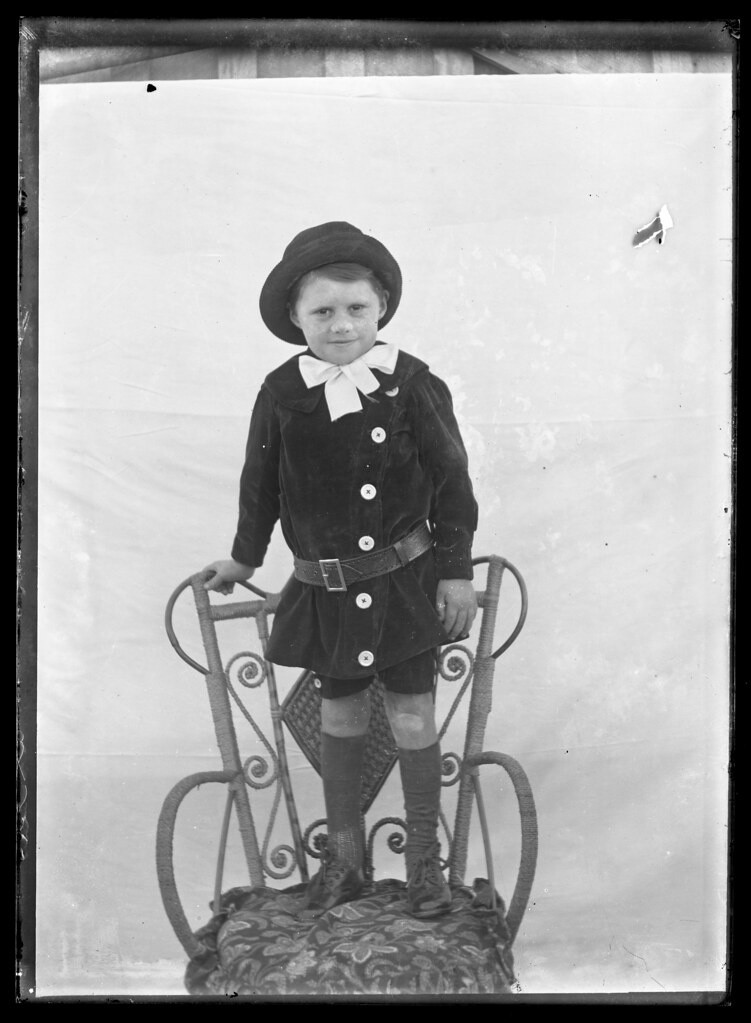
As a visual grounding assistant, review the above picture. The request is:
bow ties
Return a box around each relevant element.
[298,344,399,422]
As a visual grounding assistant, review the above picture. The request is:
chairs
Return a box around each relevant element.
[156,554,540,1003]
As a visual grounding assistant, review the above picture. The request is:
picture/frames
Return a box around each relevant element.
[18,16,737,996]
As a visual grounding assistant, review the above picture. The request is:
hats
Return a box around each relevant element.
[259,221,402,346]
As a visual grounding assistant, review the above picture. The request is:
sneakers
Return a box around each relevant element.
[293,839,365,922]
[404,841,452,920]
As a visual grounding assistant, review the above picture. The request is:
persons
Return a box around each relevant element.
[201,221,478,919]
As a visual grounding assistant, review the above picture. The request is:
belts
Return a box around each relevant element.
[293,520,435,592]
